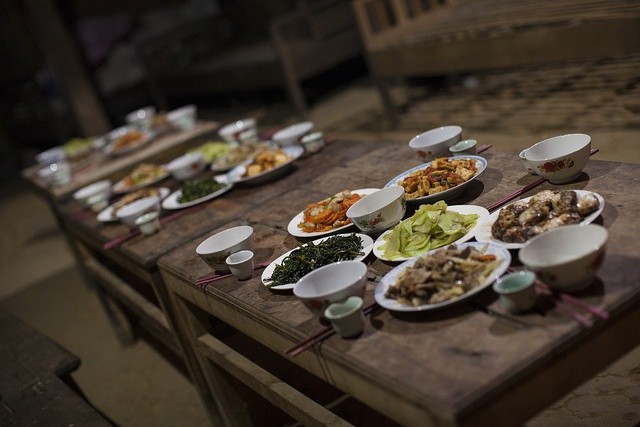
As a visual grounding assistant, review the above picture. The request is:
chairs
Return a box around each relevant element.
[144,1,364,120]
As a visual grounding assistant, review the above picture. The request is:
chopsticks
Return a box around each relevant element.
[284,303,379,358]
[69,206,93,222]
[102,204,203,251]
[486,148,598,212]
[479,142,494,155]
[509,267,609,325]
[195,261,270,286]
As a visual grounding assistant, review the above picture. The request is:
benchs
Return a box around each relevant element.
[1,298,79,402]
[346,0,639,131]
[0,373,111,426]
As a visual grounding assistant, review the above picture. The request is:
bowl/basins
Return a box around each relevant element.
[217,116,259,147]
[408,124,464,164]
[345,185,406,235]
[116,197,162,231]
[525,132,591,183]
[271,120,314,147]
[195,225,253,273]
[168,151,206,181]
[293,261,370,325]
[518,225,610,294]
[33,104,199,186]
[72,180,111,212]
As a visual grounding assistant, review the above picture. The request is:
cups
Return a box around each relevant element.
[299,132,327,156]
[451,139,478,155]
[135,211,162,238]
[518,147,534,175]
[492,273,539,310]
[325,296,366,338]
[223,249,256,281]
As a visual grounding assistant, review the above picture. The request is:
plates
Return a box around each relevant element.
[373,204,489,261]
[383,155,489,205]
[229,145,304,186]
[260,234,374,291]
[161,175,233,210]
[286,187,380,238]
[111,163,170,194]
[476,187,606,251]
[374,240,512,312]
